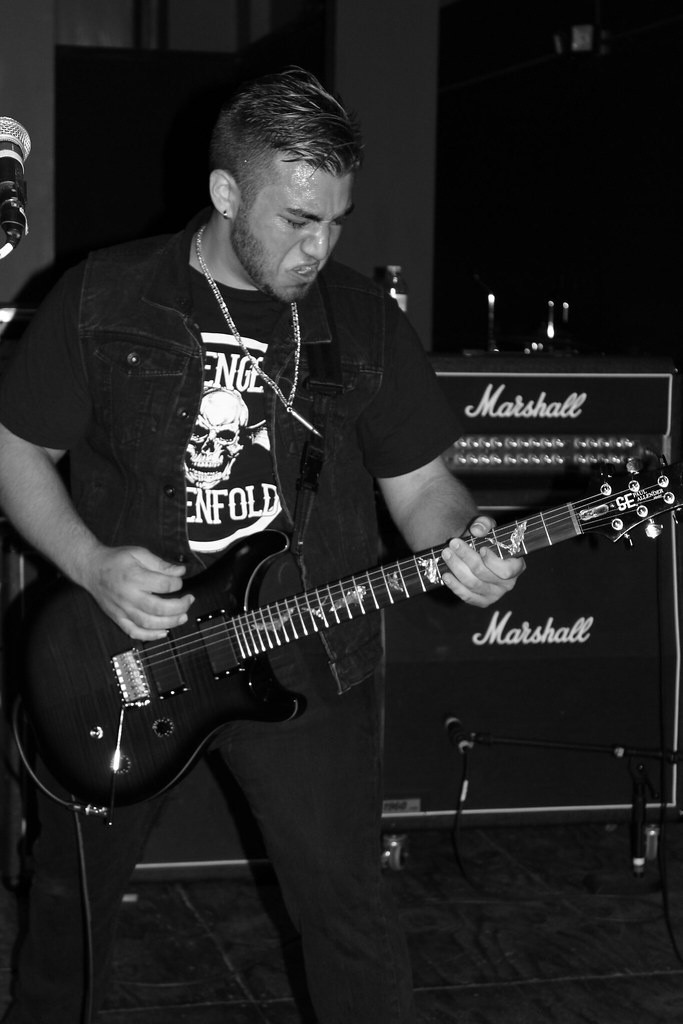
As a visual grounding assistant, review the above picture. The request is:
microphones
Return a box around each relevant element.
[442,712,473,753]
[632,780,647,878]
[0,116,32,243]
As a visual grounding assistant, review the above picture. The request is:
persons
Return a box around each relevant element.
[2,72,531,1022]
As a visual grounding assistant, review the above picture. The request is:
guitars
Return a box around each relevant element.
[1,456,682,812]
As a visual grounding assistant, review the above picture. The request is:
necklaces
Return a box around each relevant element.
[193,225,323,439]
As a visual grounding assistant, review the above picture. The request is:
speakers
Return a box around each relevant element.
[373,482,682,829]
